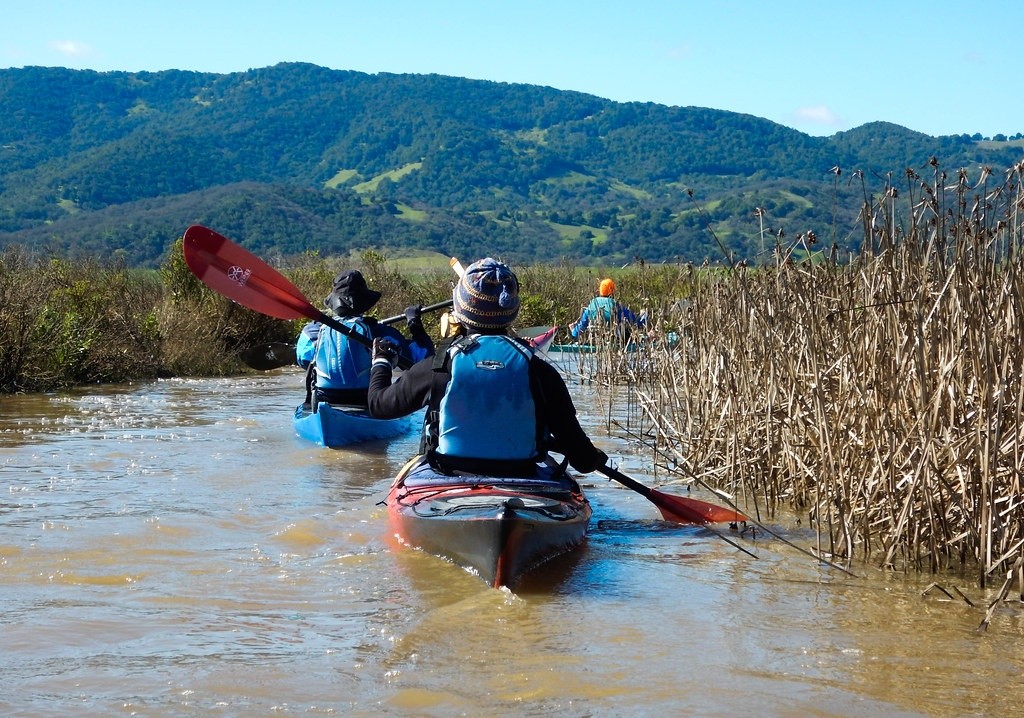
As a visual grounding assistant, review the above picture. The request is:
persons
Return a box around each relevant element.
[294,270,437,415]
[367,255,609,475]
[567,277,645,345]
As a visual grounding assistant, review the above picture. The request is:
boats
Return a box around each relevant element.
[293,401,429,449]
[388,454,593,589]
[547,331,680,354]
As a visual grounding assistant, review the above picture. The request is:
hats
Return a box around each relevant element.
[453,256,519,333]
[325,269,381,316]
[600,279,617,296]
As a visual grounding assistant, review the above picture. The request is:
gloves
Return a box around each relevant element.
[404,305,421,325]
[371,336,402,372]
[581,447,607,473]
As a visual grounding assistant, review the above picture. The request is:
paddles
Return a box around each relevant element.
[450,257,466,278]
[181,224,749,523]
[239,299,453,371]
